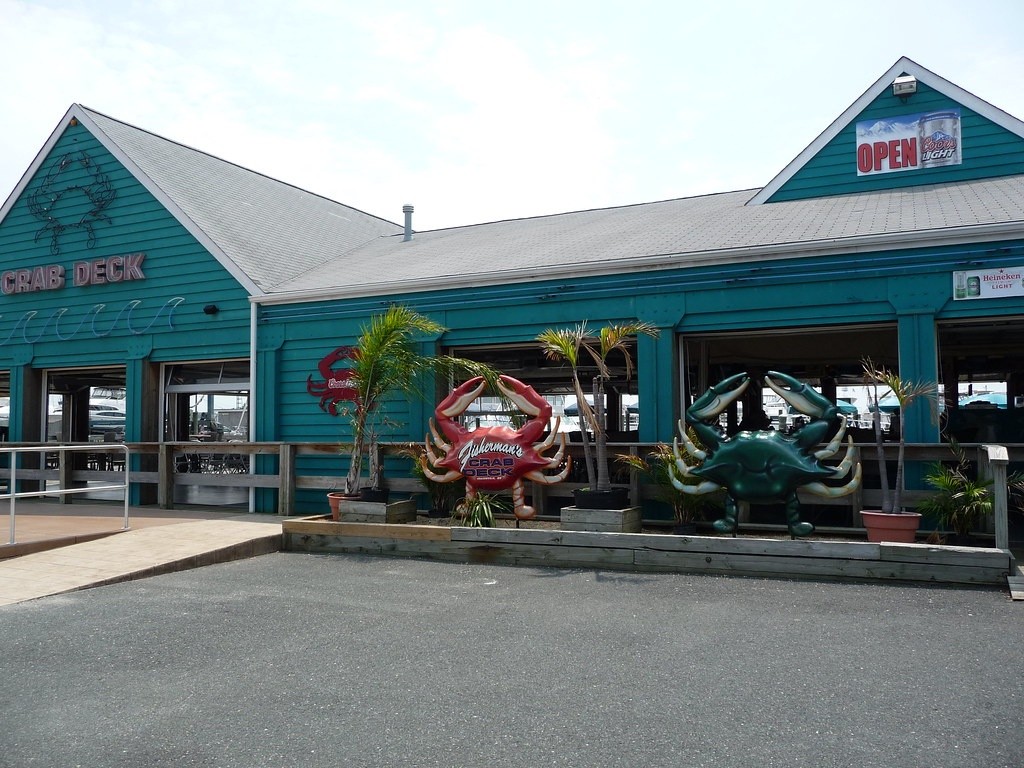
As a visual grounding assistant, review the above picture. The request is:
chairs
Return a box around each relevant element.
[46,432,248,474]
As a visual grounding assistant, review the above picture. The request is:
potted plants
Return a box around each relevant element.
[535,317,662,510]
[859,354,950,544]
[326,301,520,522]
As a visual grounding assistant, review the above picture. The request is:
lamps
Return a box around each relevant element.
[203,304,220,315]
[892,75,917,103]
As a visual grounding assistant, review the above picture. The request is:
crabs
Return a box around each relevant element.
[666,370,862,537]
[307,346,374,416]
[422,374,572,519]
